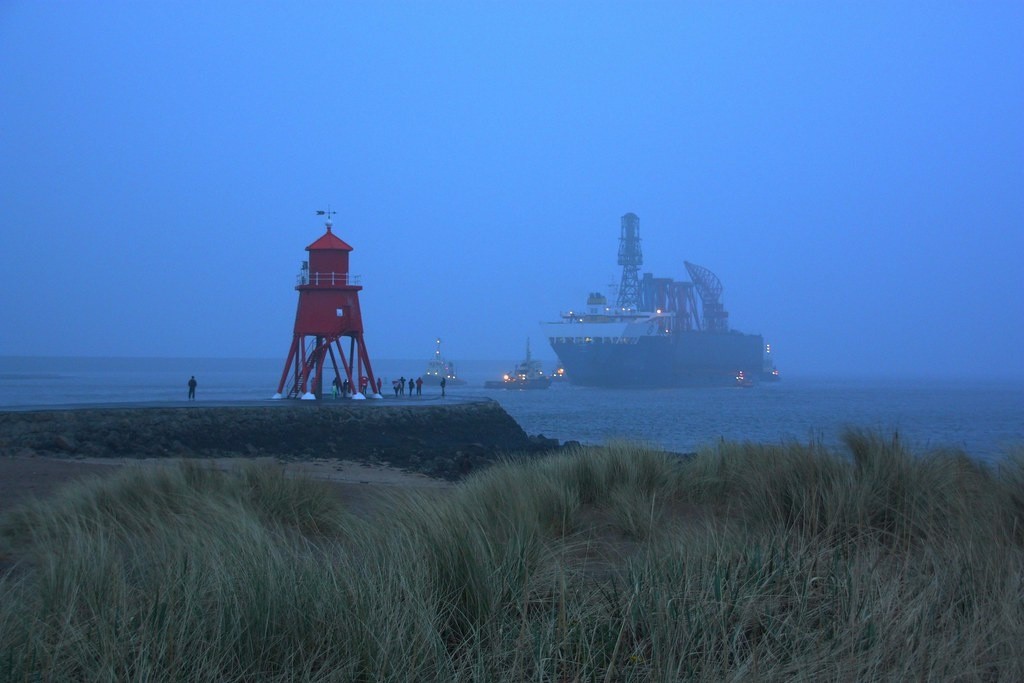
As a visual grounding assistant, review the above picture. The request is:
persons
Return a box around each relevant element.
[416,377,423,395]
[188,376,197,399]
[333,377,340,395]
[408,379,414,396]
[393,377,406,396]
[362,377,368,394]
[377,378,382,394]
[441,378,446,396]
[343,379,349,393]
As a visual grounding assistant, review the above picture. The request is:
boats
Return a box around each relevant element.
[551,359,567,381]
[423,339,467,386]
[505,336,548,389]
[539,213,781,386]
